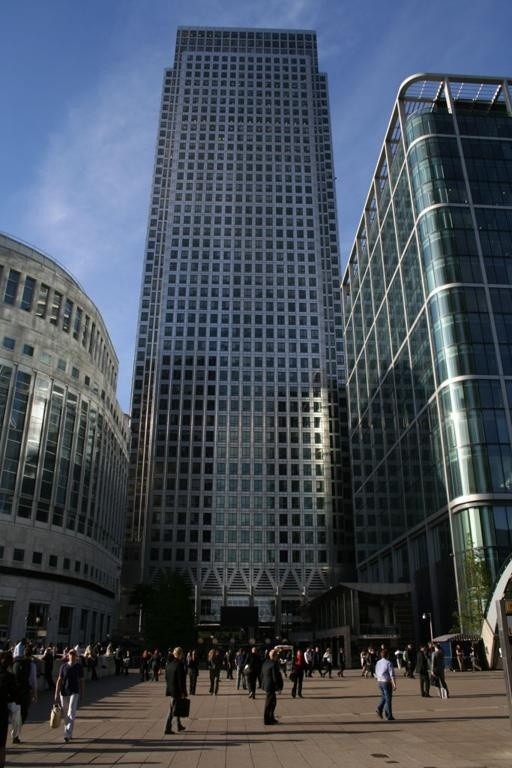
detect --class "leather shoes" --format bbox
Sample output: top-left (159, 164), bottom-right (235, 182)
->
top-left (165, 729), bottom-right (175, 734)
top-left (177, 725), bottom-right (186, 732)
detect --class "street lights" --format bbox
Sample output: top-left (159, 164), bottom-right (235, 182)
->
top-left (137, 600), bottom-right (145, 631)
top-left (421, 611), bottom-right (434, 644)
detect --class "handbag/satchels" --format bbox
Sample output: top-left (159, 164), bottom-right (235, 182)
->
top-left (172, 696), bottom-right (190, 718)
top-left (430, 674), bottom-right (439, 687)
top-left (49, 703), bottom-right (62, 729)
